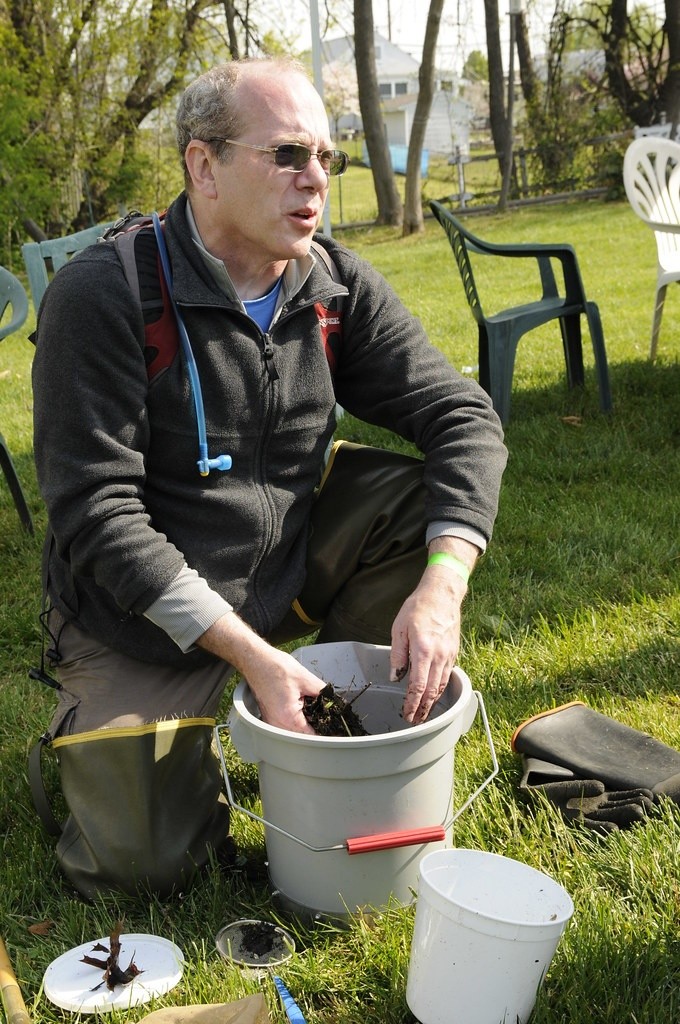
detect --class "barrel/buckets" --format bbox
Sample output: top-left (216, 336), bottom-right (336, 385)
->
top-left (215, 641), bottom-right (499, 913)
top-left (406, 849), bottom-right (574, 1024)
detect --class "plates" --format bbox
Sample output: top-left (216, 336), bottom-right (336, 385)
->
top-left (43, 933), bottom-right (185, 1014)
top-left (214, 919), bottom-right (296, 966)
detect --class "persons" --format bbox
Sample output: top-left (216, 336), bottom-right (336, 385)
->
top-left (25, 54), bottom-right (511, 910)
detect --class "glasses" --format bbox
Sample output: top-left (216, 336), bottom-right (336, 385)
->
top-left (205, 135), bottom-right (352, 178)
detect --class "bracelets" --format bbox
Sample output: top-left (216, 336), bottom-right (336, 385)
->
top-left (427, 552), bottom-right (470, 585)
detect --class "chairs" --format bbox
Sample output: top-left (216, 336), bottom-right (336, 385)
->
top-left (621, 135), bottom-right (680, 364)
top-left (1, 268), bottom-right (37, 542)
top-left (427, 197), bottom-right (613, 432)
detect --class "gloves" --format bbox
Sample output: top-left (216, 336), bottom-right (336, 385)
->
top-left (511, 701), bottom-right (680, 810)
top-left (517, 752), bottom-right (656, 842)
